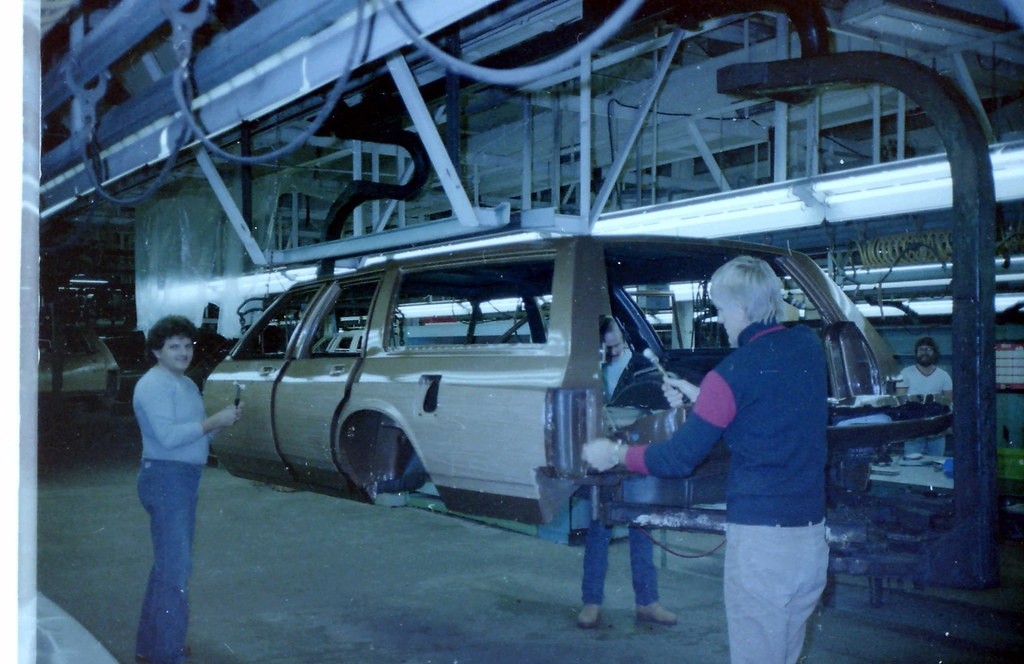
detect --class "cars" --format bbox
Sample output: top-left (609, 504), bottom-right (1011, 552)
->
top-left (205, 231), bottom-right (900, 539)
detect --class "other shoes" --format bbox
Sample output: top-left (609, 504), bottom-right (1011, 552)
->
top-left (578, 604), bottom-right (599, 628)
top-left (636, 602), bottom-right (679, 625)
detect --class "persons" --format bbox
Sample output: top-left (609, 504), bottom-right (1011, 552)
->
top-left (133, 315), bottom-right (243, 664)
top-left (578, 317), bottom-right (677, 628)
top-left (895, 337), bottom-right (953, 457)
top-left (581, 254), bottom-right (830, 664)
